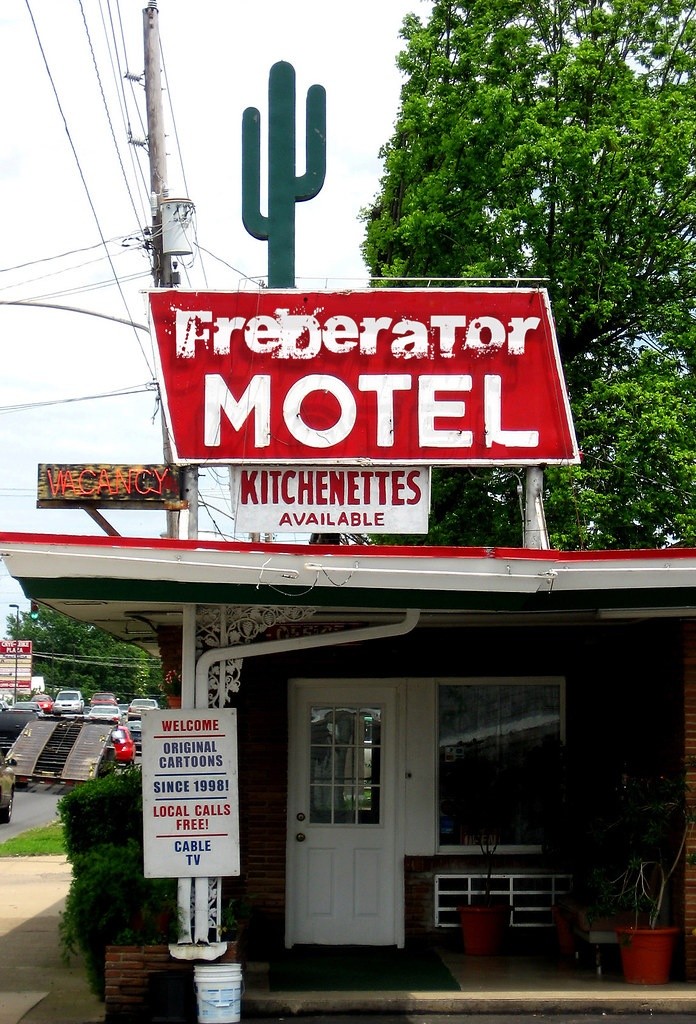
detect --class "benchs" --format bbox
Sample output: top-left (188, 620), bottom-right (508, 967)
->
top-left (557, 862), bottom-right (659, 934)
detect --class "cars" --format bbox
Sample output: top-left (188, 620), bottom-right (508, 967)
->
top-left (88, 693), bottom-right (120, 706)
top-left (127, 699), bottom-right (160, 721)
top-left (0, 701), bottom-right (10, 712)
top-left (52, 691), bottom-right (85, 715)
top-left (29, 694), bottom-right (54, 713)
top-left (11, 702), bottom-right (44, 716)
top-left (111, 727), bottom-right (138, 768)
top-left (125, 720), bottom-right (142, 752)
top-left (89, 706), bottom-right (123, 726)
top-left (117, 703), bottom-right (130, 715)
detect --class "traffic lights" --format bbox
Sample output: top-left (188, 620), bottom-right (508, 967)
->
top-left (30, 600), bottom-right (39, 621)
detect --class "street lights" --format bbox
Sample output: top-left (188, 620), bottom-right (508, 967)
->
top-left (8, 604), bottom-right (20, 705)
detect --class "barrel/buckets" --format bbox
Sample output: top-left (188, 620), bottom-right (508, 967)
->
top-left (194, 962), bottom-right (243, 1023)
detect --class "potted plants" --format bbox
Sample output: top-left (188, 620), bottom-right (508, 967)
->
top-left (453, 832), bottom-right (515, 956)
top-left (599, 753), bottom-right (696, 985)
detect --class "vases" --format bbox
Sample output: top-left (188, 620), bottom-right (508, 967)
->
top-left (167, 697), bottom-right (180, 709)
top-left (552, 906), bottom-right (576, 955)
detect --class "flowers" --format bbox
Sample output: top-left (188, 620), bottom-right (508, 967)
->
top-left (157, 670), bottom-right (182, 696)
